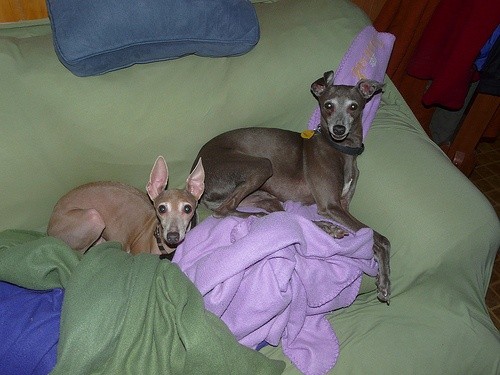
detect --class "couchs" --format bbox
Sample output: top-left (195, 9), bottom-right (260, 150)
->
top-left (0, 0), bottom-right (500, 375)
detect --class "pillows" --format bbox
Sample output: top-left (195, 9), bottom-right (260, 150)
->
top-left (45, 0), bottom-right (260, 77)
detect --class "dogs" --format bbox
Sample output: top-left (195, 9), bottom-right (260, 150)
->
top-left (189, 70), bottom-right (392, 306)
top-left (46, 154), bottom-right (206, 255)
